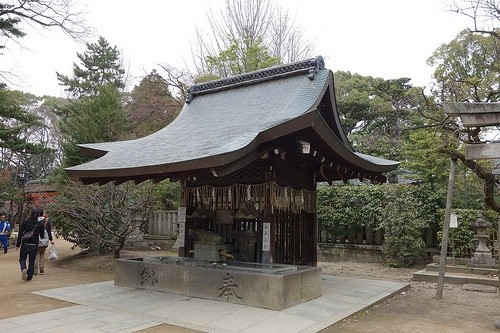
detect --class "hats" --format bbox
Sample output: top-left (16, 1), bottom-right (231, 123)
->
top-left (32, 209), bottom-right (40, 215)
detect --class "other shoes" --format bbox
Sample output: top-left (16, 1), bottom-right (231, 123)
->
top-left (40, 267), bottom-right (44, 273)
top-left (22, 269), bottom-right (27, 280)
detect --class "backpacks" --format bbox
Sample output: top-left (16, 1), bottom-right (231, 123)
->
top-left (38, 229), bottom-right (49, 247)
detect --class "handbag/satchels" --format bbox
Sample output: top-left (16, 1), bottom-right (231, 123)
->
top-left (22, 223), bottom-right (38, 242)
top-left (48, 245), bottom-right (58, 265)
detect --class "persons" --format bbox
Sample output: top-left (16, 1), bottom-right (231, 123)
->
top-left (0, 209), bottom-right (55, 280)
